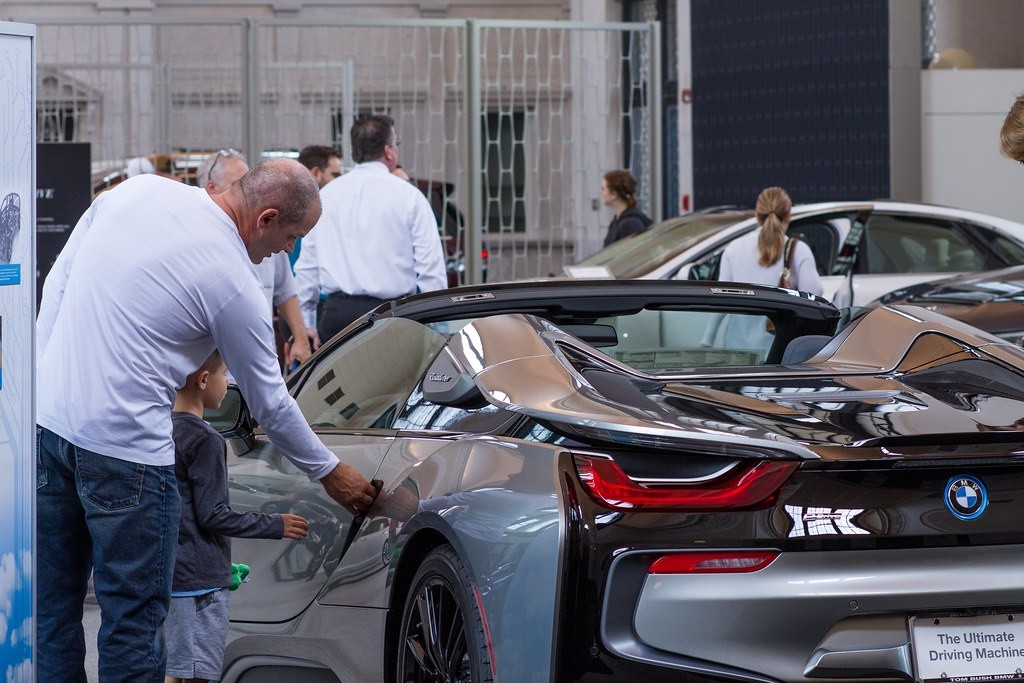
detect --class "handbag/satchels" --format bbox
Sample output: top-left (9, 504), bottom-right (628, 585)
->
top-left (766, 237), bottom-right (801, 336)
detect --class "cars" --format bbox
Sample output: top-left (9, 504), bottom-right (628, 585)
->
top-left (851, 266), bottom-right (1024, 350)
top-left (554, 200), bottom-right (1024, 300)
top-left (224, 475), bottom-right (292, 514)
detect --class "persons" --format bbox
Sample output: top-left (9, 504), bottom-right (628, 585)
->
top-left (601, 170), bottom-right (654, 248)
top-left (35, 115), bottom-right (449, 683)
top-left (701, 187), bottom-right (824, 364)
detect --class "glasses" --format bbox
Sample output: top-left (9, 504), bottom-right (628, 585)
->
top-left (396, 137), bottom-right (399, 145)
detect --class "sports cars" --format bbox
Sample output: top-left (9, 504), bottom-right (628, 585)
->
top-left (202, 279), bottom-right (1024, 683)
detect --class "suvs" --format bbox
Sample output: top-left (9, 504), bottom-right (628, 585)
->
top-left (88, 147), bottom-right (490, 288)
